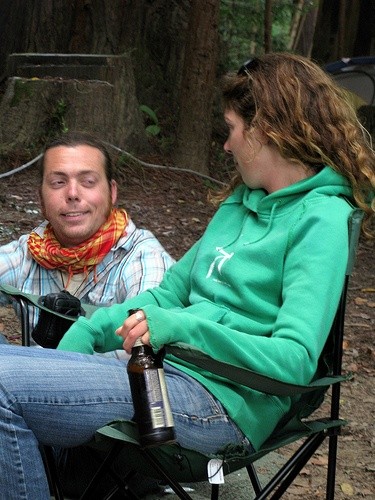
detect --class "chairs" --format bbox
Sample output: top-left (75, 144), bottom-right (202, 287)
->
top-left (0, 207), bottom-right (366, 500)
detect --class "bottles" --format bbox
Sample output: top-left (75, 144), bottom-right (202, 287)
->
top-left (128, 308), bottom-right (176, 449)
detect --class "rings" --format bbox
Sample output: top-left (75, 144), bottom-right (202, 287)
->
top-left (135, 312), bottom-right (143, 322)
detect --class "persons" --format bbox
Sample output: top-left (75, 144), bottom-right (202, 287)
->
top-left (0, 131), bottom-right (176, 348)
top-left (0, 52), bottom-right (375, 500)
